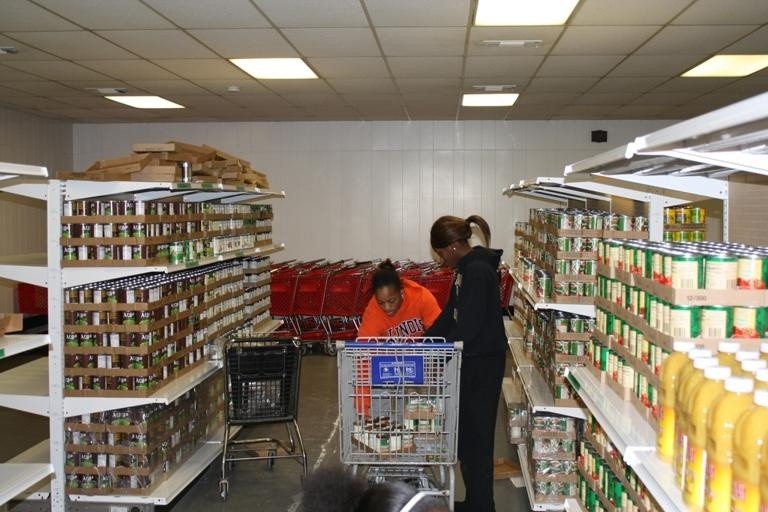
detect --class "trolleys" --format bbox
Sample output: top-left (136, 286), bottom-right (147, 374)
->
top-left (212, 327), bottom-right (316, 500)
top-left (256, 256), bottom-right (514, 357)
top-left (330, 331), bottom-right (469, 508)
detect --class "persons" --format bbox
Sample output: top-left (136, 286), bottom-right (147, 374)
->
top-left (353, 263), bottom-right (442, 415)
top-left (420, 215), bottom-right (507, 512)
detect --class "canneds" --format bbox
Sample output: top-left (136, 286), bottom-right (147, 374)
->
top-left (62, 197), bottom-right (281, 265)
top-left (662, 204), bottom-right (704, 244)
top-left (506, 206), bottom-right (647, 406)
top-left (62, 253), bottom-right (275, 395)
top-left (62, 368), bottom-right (226, 491)
top-left (351, 399), bottom-right (443, 457)
top-left (587, 240), bottom-right (768, 416)
top-left (510, 408), bottom-right (576, 503)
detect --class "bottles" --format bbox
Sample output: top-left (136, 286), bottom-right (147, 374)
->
top-left (656, 340), bottom-right (768, 512)
